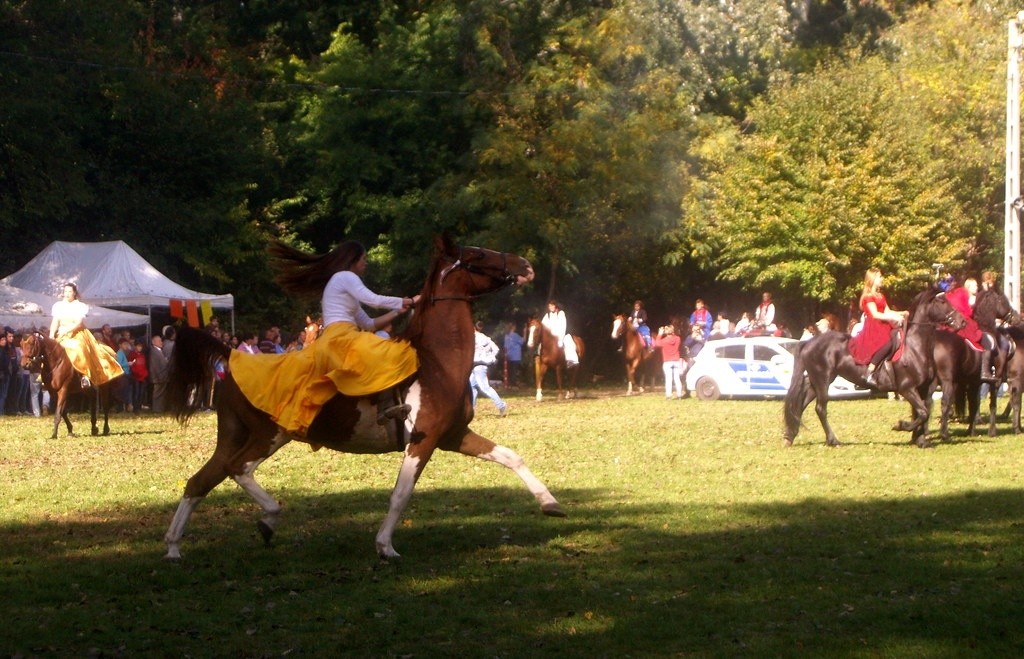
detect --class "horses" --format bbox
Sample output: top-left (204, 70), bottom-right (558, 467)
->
top-left (923, 315), bottom-right (1024, 438)
top-left (611, 312), bottom-right (664, 393)
top-left (668, 312), bottom-right (692, 338)
top-left (163, 232), bottom-right (568, 561)
top-left (19, 330), bottom-right (112, 439)
top-left (527, 317), bottom-right (586, 400)
top-left (783, 282), bottom-right (967, 448)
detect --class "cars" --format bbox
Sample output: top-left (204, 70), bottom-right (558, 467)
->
top-left (685, 335), bottom-right (871, 401)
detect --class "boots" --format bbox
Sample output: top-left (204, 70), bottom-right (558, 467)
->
top-left (979, 349), bottom-right (998, 381)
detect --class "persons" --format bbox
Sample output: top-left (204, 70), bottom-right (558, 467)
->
top-left (0, 326), bottom-right (177, 417)
top-left (49, 283), bottom-right (125, 388)
top-left (654, 325), bottom-right (684, 400)
top-left (617, 299), bottom-right (653, 353)
top-left (320, 238), bottom-right (420, 426)
top-left (469, 321), bottom-right (506, 418)
top-left (375, 322), bottom-right (393, 339)
top-left (683, 266), bottom-right (1001, 384)
top-left (505, 321), bottom-right (528, 389)
top-left (541, 300), bottom-right (579, 368)
top-left (208, 312), bottom-right (325, 405)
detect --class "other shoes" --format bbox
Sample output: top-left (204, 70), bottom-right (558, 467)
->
top-left (42, 405), bottom-right (49, 416)
top-left (115, 407), bottom-right (125, 414)
top-left (567, 361), bottom-right (574, 368)
top-left (861, 374), bottom-right (878, 385)
top-left (500, 402), bottom-right (507, 416)
top-left (10, 409), bottom-right (34, 416)
top-left (133, 407), bottom-right (140, 415)
top-left (377, 403), bottom-right (412, 425)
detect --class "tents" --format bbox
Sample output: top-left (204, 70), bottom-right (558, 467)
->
top-left (0, 240), bottom-right (235, 348)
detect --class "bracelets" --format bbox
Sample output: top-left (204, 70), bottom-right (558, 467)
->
top-left (410, 296), bottom-right (414, 306)
